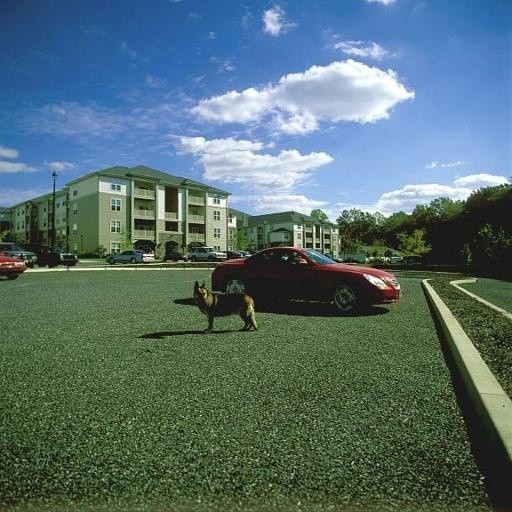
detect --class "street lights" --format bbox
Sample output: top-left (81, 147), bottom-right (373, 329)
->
top-left (381, 239), bottom-right (387, 255)
top-left (51, 171), bottom-right (59, 244)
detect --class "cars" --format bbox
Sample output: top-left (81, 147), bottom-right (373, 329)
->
top-left (106, 250), bottom-right (154, 264)
top-left (238, 251), bottom-right (252, 258)
top-left (1, 243), bottom-right (38, 269)
top-left (225, 250), bottom-right (243, 260)
top-left (211, 247), bottom-right (401, 312)
top-left (332, 255), bottom-right (400, 263)
top-left (164, 252), bottom-right (188, 262)
top-left (0, 251), bottom-right (27, 279)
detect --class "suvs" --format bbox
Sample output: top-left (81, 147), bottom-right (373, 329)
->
top-left (189, 247), bottom-right (227, 262)
top-left (23, 244), bottom-right (79, 268)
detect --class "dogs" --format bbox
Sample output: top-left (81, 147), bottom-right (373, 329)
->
top-left (194, 280), bottom-right (257, 333)
